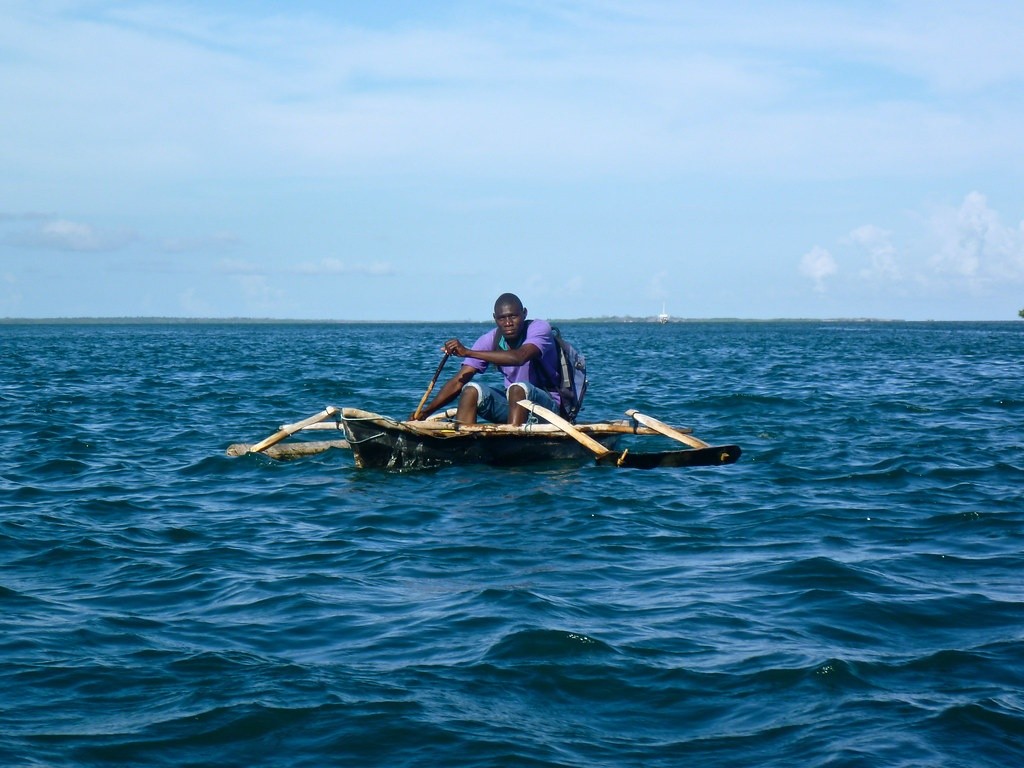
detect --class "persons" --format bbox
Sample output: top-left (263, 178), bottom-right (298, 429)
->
top-left (407, 293), bottom-right (565, 426)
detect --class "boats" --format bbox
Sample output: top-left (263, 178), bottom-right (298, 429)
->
top-left (226, 399), bottom-right (742, 475)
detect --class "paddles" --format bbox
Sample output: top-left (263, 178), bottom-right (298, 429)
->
top-left (413, 352), bottom-right (449, 420)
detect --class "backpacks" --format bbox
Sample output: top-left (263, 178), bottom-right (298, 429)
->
top-left (493, 319), bottom-right (589, 424)
